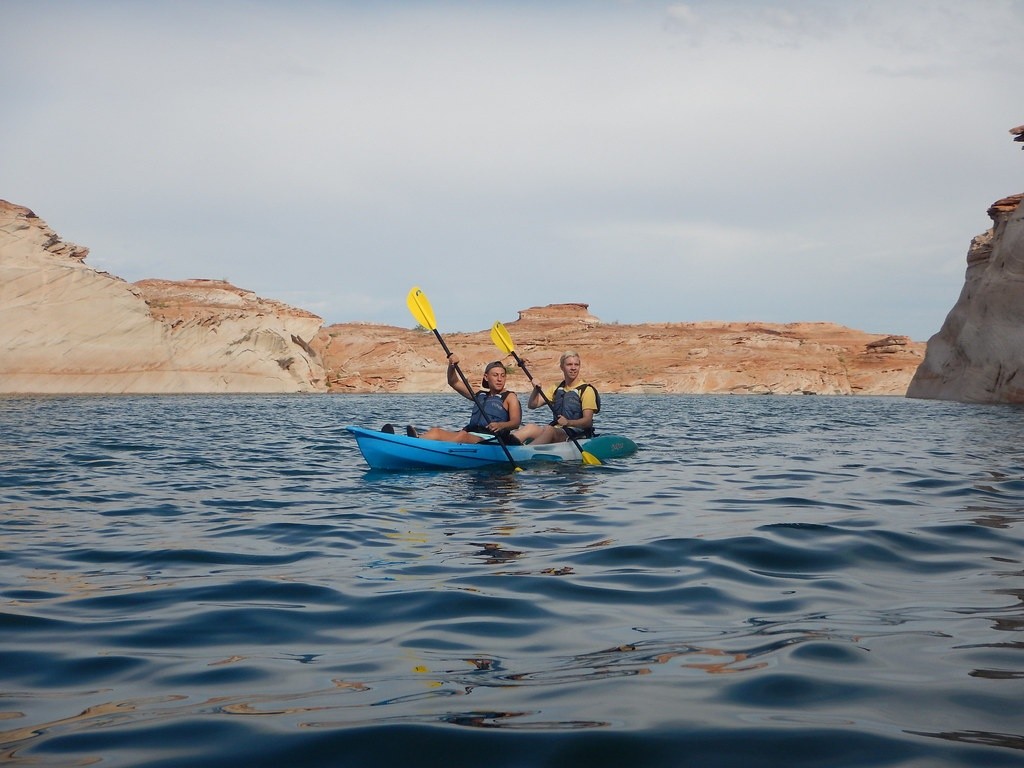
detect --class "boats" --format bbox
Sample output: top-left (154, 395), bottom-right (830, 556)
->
top-left (346, 424), bottom-right (640, 474)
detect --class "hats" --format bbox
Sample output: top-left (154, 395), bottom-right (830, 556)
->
top-left (482, 361), bottom-right (506, 389)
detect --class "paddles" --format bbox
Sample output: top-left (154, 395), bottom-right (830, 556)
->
top-left (490, 318), bottom-right (602, 465)
top-left (407, 286), bottom-right (526, 472)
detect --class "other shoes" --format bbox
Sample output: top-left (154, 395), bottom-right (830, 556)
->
top-left (381, 423), bottom-right (394, 434)
top-left (407, 425), bottom-right (419, 438)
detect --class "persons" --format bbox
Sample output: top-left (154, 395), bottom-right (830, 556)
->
top-left (509, 350), bottom-right (601, 445)
top-left (381, 353), bottom-right (522, 444)
